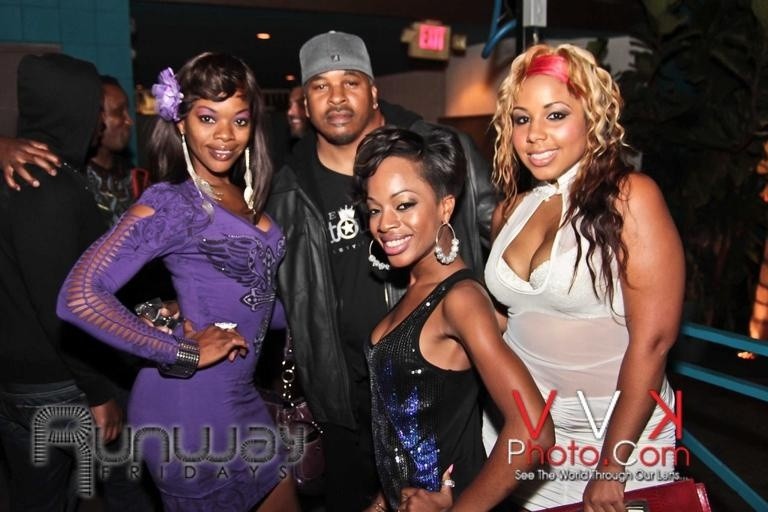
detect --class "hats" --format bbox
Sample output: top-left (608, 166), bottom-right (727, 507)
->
top-left (299, 30), bottom-right (374, 87)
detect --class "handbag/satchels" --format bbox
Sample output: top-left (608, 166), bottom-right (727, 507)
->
top-left (257, 327), bottom-right (326, 487)
top-left (534, 478), bottom-right (712, 512)
